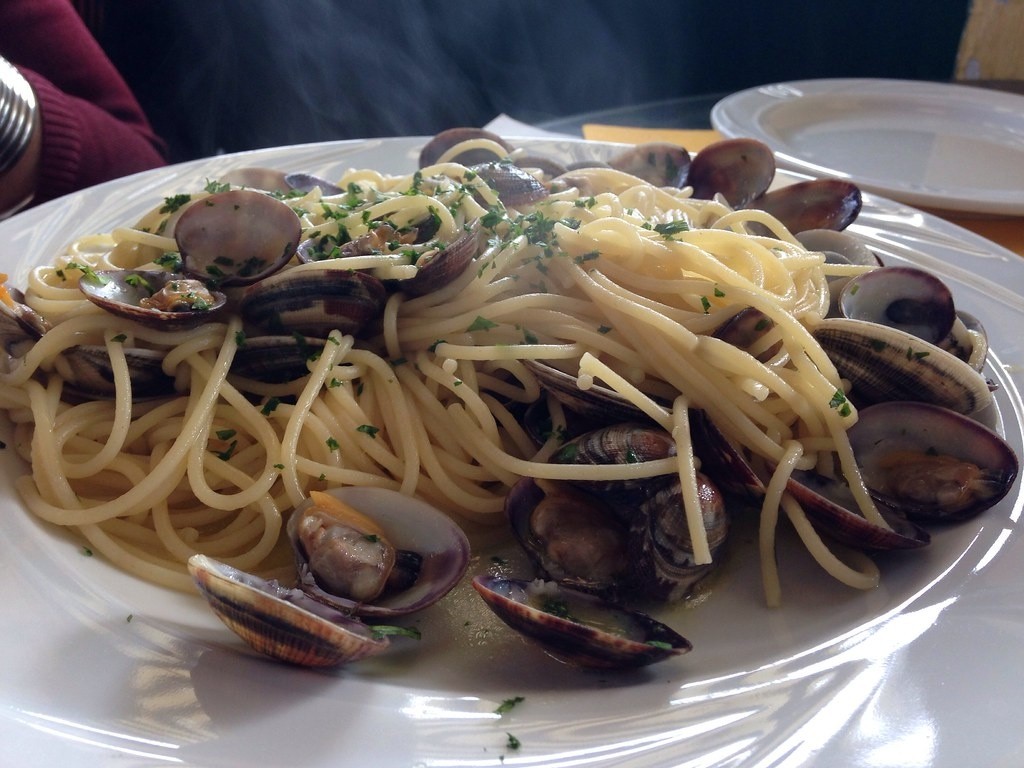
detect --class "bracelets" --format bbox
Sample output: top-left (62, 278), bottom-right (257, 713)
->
top-left (0, 54), bottom-right (37, 174)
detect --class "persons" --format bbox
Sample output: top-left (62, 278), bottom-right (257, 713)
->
top-left (0, 0), bottom-right (171, 225)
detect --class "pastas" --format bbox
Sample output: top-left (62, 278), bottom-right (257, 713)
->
top-left (0, 138), bottom-right (1023, 608)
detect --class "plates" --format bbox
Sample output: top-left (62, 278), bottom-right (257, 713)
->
top-left (0, 135), bottom-right (1024, 768)
top-left (710, 78), bottom-right (1024, 220)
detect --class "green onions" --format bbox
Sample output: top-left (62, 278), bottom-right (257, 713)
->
top-left (58, 155), bottom-right (929, 750)
